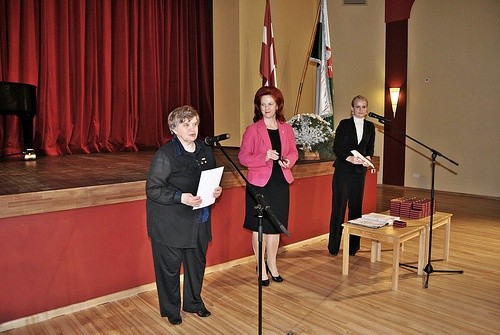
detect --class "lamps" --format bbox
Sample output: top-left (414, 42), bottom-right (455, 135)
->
top-left (390, 88), bottom-right (400, 117)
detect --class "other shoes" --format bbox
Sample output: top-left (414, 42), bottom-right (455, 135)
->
top-left (329, 249), bottom-right (338, 255)
top-left (349, 251), bottom-right (356, 255)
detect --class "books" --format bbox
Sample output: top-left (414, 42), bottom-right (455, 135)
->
top-left (347, 213), bottom-right (401, 229)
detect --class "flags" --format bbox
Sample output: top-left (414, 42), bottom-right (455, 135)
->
top-left (260, 0), bottom-right (278, 87)
top-left (309, 0), bottom-right (334, 131)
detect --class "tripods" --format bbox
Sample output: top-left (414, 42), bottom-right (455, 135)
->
top-left (379, 119), bottom-right (465, 288)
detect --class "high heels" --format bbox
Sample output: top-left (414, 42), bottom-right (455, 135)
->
top-left (265, 261), bottom-right (283, 282)
top-left (256, 266), bottom-right (269, 286)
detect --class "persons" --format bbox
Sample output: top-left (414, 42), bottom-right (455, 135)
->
top-left (145, 106), bottom-right (222, 325)
top-left (328, 95), bottom-right (376, 256)
top-left (237, 86), bottom-right (299, 286)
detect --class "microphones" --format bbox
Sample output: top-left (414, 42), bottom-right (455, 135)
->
top-left (205, 133), bottom-right (231, 145)
top-left (369, 112), bottom-right (392, 123)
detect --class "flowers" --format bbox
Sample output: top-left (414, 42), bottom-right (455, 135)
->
top-left (286, 113), bottom-right (334, 152)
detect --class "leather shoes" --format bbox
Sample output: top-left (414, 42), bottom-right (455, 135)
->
top-left (168, 316), bottom-right (182, 325)
top-left (196, 309), bottom-right (211, 316)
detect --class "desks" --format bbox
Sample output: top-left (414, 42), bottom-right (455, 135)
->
top-left (341, 210), bottom-right (453, 291)
